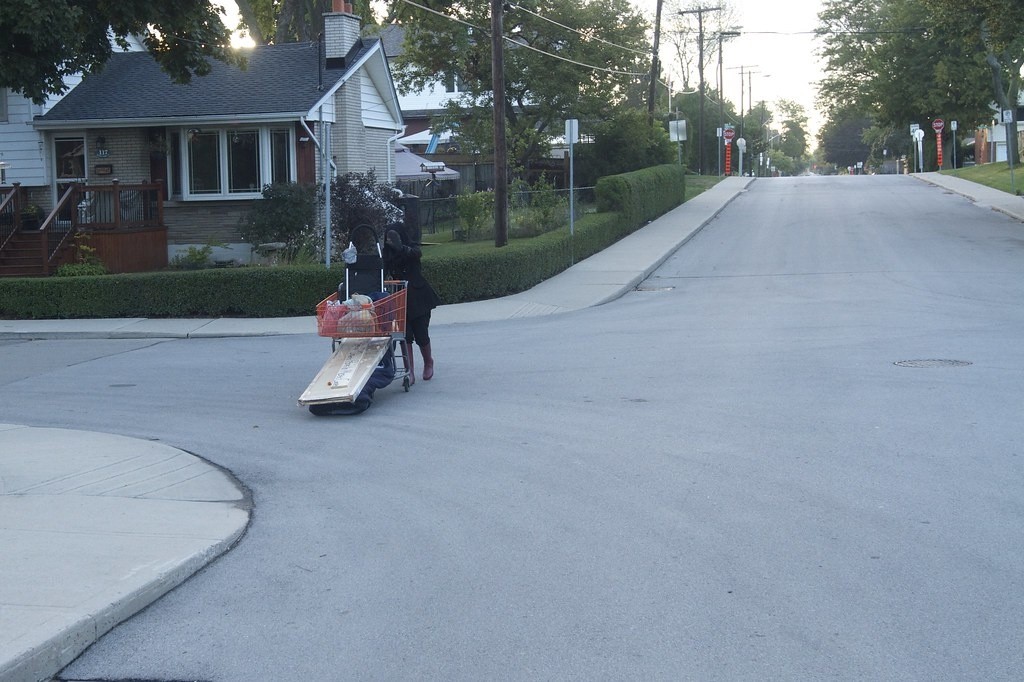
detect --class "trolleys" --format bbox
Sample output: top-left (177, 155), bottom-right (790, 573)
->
top-left (315, 278), bottom-right (412, 392)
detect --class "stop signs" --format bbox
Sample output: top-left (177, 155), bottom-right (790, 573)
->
top-left (932, 119), bottom-right (944, 132)
top-left (724, 129), bottom-right (735, 139)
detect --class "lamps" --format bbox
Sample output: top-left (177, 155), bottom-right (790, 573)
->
top-left (95, 135), bottom-right (106, 149)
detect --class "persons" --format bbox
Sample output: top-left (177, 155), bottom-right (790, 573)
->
top-left (382, 224), bottom-right (439, 387)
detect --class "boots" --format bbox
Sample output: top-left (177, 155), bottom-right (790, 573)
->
top-left (400, 343), bottom-right (415, 387)
top-left (419, 343), bottom-right (433, 380)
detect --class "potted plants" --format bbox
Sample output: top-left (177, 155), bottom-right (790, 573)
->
top-left (19, 203), bottom-right (45, 223)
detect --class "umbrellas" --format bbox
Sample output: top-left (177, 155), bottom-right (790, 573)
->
top-left (395, 141), bottom-right (459, 181)
top-left (398, 122), bottom-right (461, 144)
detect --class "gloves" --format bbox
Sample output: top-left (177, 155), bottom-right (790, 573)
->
top-left (386, 229), bottom-right (404, 252)
